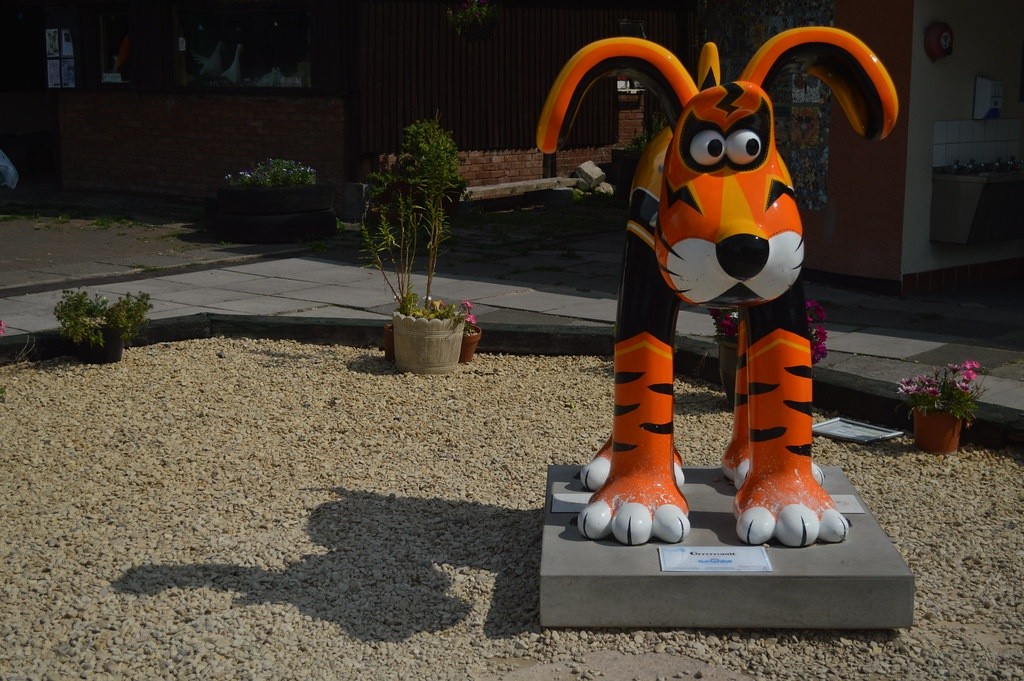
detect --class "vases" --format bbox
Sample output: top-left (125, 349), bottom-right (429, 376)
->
top-left (382, 325), bottom-right (395, 362)
top-left (460, 325), bottom-right (481, 362)
top-left (913, 409), bottom-right (962, 453)
top-left (716, 339), bottom-right (738, 405)
top-left (464, 22), bottom-right (494, 40)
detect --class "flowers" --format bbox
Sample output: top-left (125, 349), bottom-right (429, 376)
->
top-left (709, 299), bottom-right (827, 365)
top-left (445, 0), bottom-right (500, 36)
top-left (460, 301), bottom-right (477, 334)
top-left (895, 360), bottom-right (988, 427)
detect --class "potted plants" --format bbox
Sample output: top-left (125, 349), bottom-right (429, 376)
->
top-left (53, 290), bottom-right (153, 365)
top-left (360, 120), bottom-right (465, 376)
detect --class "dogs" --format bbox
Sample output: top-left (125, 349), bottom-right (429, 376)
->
top-left (534, 24), bottom-right (900, 548)
